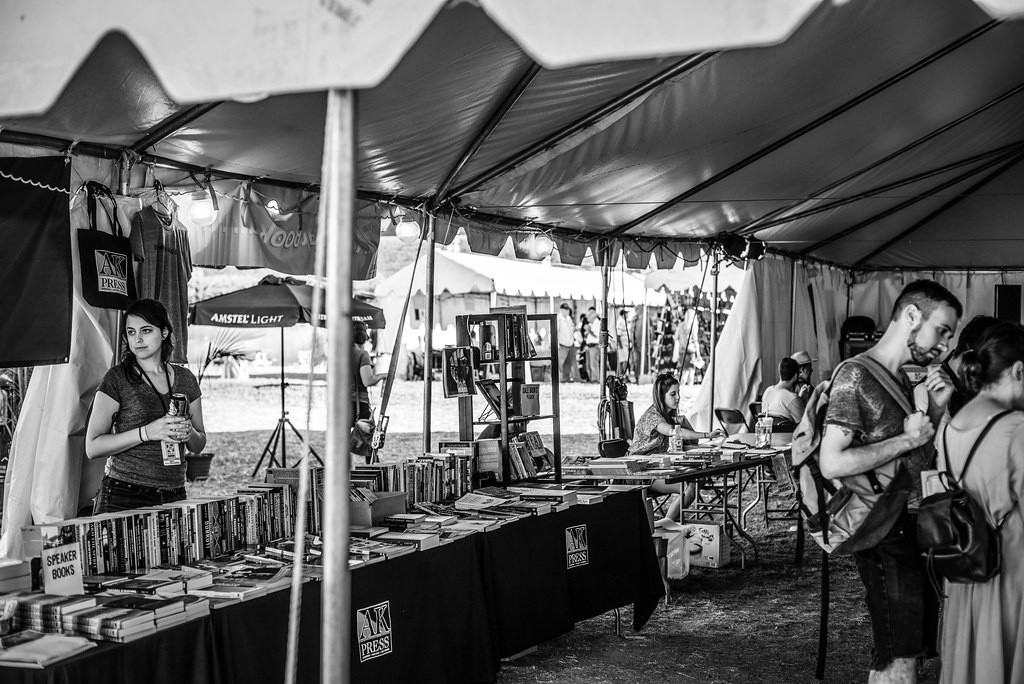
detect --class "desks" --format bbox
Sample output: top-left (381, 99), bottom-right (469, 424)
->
top-left (0, 485), bottom-right (666, 684)
top-left (557, 446), bottom-right (780, 572)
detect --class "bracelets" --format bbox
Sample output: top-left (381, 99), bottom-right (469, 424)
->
top-left (139, 426), bottom-right (144, 443)
top-left (145, 425), bottom-right (150, 441)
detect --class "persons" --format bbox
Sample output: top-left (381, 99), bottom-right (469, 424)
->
top-left (349, 320), bottom-right (394, 464)
top-left (762, 350), bottom-right (815, 425)
top-left (674, 308), bottom-right (700, 387)
top-left (816, 279), bottom-right (1024, 684)
top-left (85, 298), bottom-right (206, 516)
top-left (558, 303), bottom-right (629, 383)
top-left (626, 373), bottom-right (728, 521)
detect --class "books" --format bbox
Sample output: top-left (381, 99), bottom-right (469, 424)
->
top-left (0, 432), bottom-right (650, 668)
top-left (627, 439), bottom-right (793, 476)
top-left (443, 305), bottom-right (540, 420)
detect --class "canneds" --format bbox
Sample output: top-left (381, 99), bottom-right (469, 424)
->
top-left (167, 393), bottom-right (188, 417)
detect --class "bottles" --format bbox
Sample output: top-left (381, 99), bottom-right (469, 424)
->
top-left (755, 418), bottom-right (765, 448)
top-left (669, 425), bottom-right (683, 452)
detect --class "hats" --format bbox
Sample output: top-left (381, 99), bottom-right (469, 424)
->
top-left (790, 350), bottom-right (818, 366)
top-left (561, 303), bottom-right (569, 309)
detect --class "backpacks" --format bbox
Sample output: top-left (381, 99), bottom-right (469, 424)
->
top-left (790, 355), bottom-right (927, 556)
top-left (915, 411), bottom-right (1022, 584)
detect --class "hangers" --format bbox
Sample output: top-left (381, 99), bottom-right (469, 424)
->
top-left (151, 180), bottom-right (172, 215)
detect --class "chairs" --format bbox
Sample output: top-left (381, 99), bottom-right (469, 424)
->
top-left (715, 403), bottom-right (797, 496)
top-left (598, 438), bottom-right (669, 520)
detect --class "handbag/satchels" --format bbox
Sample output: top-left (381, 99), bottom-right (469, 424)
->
top-left (349, 421), bottom-right (376, 457)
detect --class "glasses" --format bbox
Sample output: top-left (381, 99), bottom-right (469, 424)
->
top-left (655, 372), bottom-right (672, 384)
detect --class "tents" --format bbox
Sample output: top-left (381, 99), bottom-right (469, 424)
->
top-left (1, 1), bottom-right (1023, 684)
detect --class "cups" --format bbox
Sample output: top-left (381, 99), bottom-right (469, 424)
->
top-left (762, 418), bottom-right (774, 446)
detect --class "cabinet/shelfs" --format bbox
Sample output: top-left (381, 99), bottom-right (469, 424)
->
top-left (456, 313), bottom-right (561, 491)
